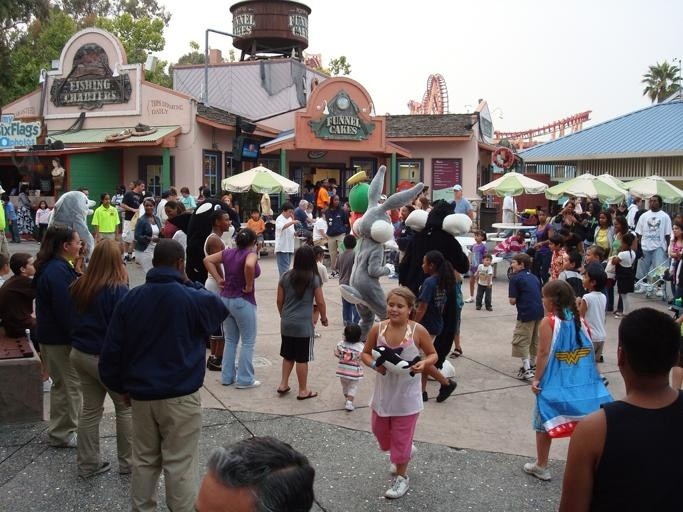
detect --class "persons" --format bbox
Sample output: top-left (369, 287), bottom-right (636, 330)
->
top-left (2, 180), bottom-right (265, 389)
top-left (494, 194), bottom-right (681, 394)
top-left (559, 307), bottom-right (681, 512)
top-left (189, 436), bottom-right (316, 512)
top-left (99, 238), bottom-right (231, 511)
top-left (524, 279), bottom-right (614, 481)
top-left (274, 176), bottom-right (494, 403)
top-left (62, 238), bottom-right (135, 479)
top-left (359, 286), bottom-right (438, 498)
top-left (32, 225), bottom-right (84, 448)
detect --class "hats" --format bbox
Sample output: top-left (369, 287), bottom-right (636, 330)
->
top-left (453, 184), bottom-right (462, 190)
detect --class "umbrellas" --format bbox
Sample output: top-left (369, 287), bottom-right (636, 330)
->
top-left (478, 171), bottom-right (626, 223)
top-left (221, 162), bottom-right (301, 216)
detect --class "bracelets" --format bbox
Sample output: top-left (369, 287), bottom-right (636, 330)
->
top-left (372, 361), bottom-right (376, 367)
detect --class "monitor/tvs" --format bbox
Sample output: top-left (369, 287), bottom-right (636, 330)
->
top-left (241, 138), bottom-right (260, 161)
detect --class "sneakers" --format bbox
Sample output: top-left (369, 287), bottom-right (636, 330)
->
top-left (436, 379), bottom-right (457, 402)
top-left (53, 434), bottom-right (80, 448)
top-left (513, 364), bottom-right (537, 380)
top-left (385, 474), bottom-right (410, 500)
top-left (206, 356), bottom-right (223, 372)
top-left (422, 390), bottom-right (429, 402)
top-left (612, 308), bottom-right (626, 321)
top-left (235, 380), bottom-right (262, 389)
top-left (464, 298), bottom-right (493, 311)
top-left (389, 445), bottom-right (417, 473)
top-left (123, 253), bottom-right (135, 263)
top-left (328, 270), bottom-right (340, 279)
top-left (523, 462), bottom-right (553, 481)
top-left (80, 461), bottom-right (110, 477)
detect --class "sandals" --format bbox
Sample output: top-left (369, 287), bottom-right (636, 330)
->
top-left (450, 347), bottom-right (463, 360)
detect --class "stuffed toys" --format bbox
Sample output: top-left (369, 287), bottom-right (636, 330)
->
top-left (48, 191), bottom-right (96, 261)
top-left (371, 346), bottom-right (420, 377)
top-left (338, 165), bottom-right (424, 342)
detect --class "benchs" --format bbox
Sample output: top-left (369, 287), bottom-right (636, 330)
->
top-left (236, 222), bottom-right (311, 259)
top-left (486, 232), bottom-right (533, 242)
top-left (487, 254), bottom-right (504, 279)
top-left (0, 325), bottom-right (44, 424)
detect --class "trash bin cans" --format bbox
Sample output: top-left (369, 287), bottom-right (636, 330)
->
top-left (480, 206), bottom-right (497, 233)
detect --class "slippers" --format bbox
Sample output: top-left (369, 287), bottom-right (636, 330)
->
top-left (277, 385), bottom-right (291, 394)
top-left (296, 390), bottom-right (318, 400)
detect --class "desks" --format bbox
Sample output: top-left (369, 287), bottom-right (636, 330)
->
top-left (492, 222), bottom-right (536, 238)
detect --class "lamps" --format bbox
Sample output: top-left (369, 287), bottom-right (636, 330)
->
top-left (362, 102), bottom-right (375, 117)
top-left (316, 100), bottom-right (331, 115)
top-left (39, 68), bottom-right (47, 84)
top-left (111, 61), bottom-right (123, 77)
